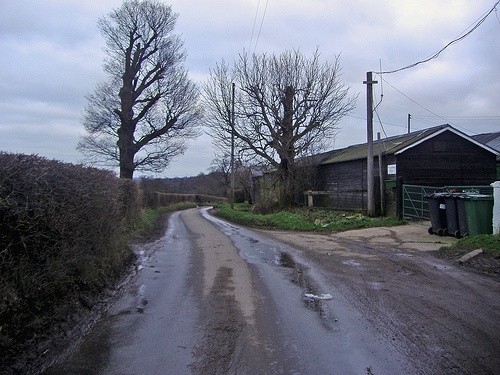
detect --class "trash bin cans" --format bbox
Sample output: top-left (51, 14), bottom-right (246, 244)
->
top-left (424, 193), bottom-right (447, 236)
top-left (463, 194), bottom-right (495, 236)
top-left (443, 193), bottom-right (462, 238)
top-left (455, 195), bottom-right (470, 237)
